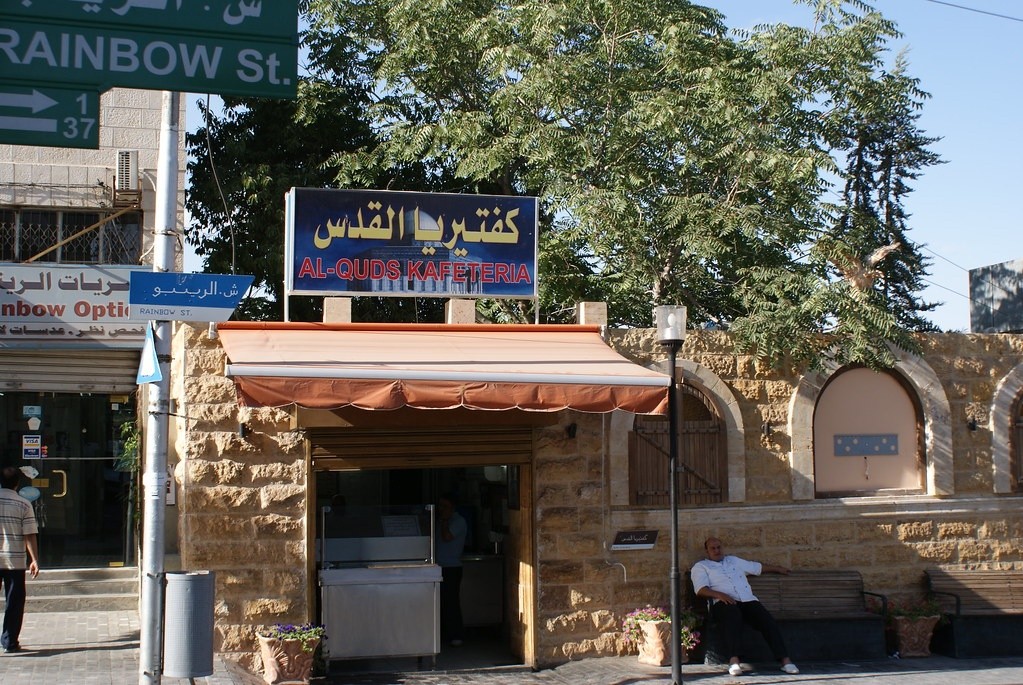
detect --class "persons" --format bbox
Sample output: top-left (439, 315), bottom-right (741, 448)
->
top-left (0, 468), bottom-right (39, 653)
top-left (690, 537), bottom-right (799, 675)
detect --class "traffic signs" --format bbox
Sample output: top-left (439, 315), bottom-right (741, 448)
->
top-left (0, 84), bottom-right (100, 150)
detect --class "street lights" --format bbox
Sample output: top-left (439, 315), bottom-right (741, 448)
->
top-left (657, 305), bottom-right (687, 685)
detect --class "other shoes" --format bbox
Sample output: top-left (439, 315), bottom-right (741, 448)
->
top-left (728, 663), bottom-right (743, 676)
top-left (780, 664), bottom-right (800, 675)
top-left (451, 639), bottom-right (464, 647)
top-left (5, 644), bottom-right (22, 653)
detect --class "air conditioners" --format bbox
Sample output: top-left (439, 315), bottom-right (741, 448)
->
top-left (116, 149), bottom-right (141, 206)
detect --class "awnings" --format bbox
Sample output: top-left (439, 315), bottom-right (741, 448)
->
top-left (216, 321), bottom-right (671, 416)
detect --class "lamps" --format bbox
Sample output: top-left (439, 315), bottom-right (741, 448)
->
top-left (967, 415), bottom-right (976, 431)
top-left (237, 423), bottom-right (252, 437)
top-left (761, 421), bottom-right (772, 437)
top-left (565, 422), bottom-right (578, 437)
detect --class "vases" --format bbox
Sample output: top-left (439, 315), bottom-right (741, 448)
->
top-left (892, 615), bottom-right (940, 659)
top-left (636, 618), bottom-right (691, 666)
top-left (259, 631), bottom-right (319, 685)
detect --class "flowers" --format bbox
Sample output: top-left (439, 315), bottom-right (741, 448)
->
top-left (269, 621), bottom-right (330, 643)
top-left (619, 602), bottom-right (698, 652)
top-left (865, 596), bottom-right (952, 627)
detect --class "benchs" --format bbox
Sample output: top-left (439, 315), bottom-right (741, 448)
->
top-left (684, 568), bottom-right (890, 667)
top-left (923, 570), bottom-right (1023, 657)
